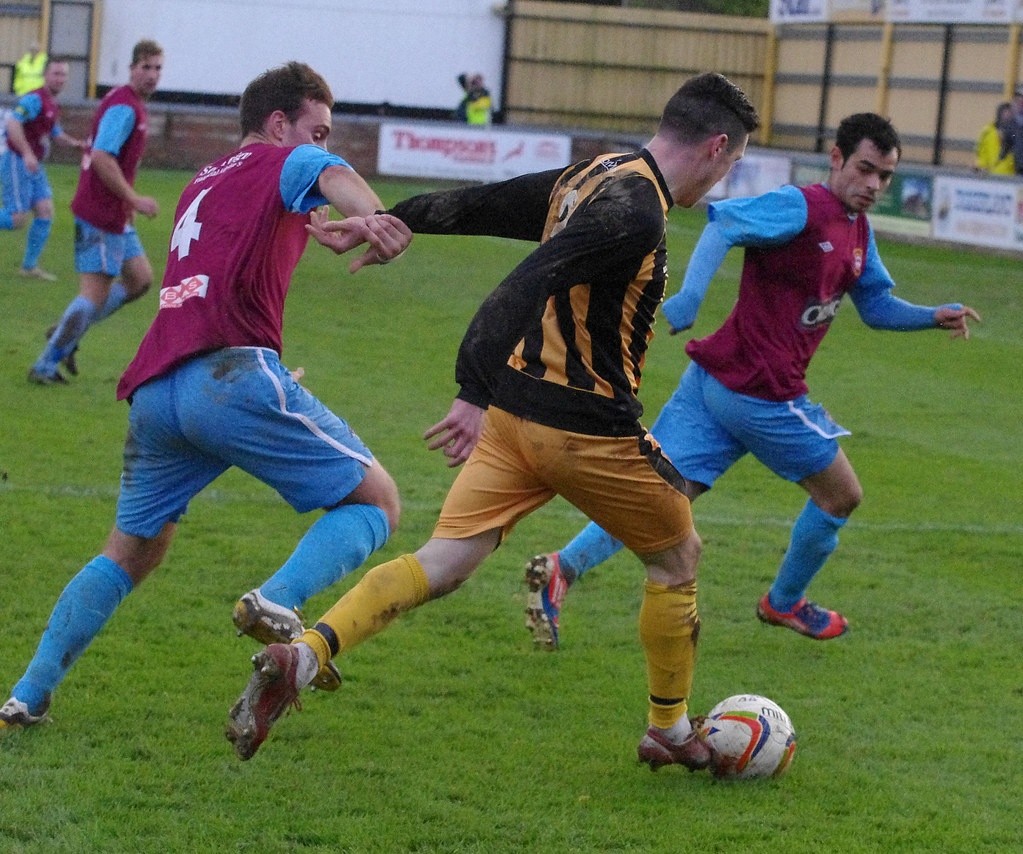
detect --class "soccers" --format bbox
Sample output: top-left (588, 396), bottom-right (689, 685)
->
top-left (698, 694), bottom-right (799, 782)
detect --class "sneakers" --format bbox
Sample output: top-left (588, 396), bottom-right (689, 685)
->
top-left (232, 587), bottom-right (342, 692)
top-left (757, 590), bottom-right (847, 640)
top-left (0, 696), bottom-right (50, 733)
top-left (225, 642), bottom-right (301, 760)
top-left (637, 722), bottom-right (712, 772)
top-left (526, 552), bottom-right (573, 651)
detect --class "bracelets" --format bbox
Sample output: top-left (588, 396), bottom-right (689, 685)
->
top-left (374, 251), bottom-right (390, 263)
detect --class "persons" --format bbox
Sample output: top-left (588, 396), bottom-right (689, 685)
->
top-left (452, 74), bottom-right (493, 124)
top-left (223, 70), bottom-right (763, 775)
top-left (0, 62), bottom-right (415, 731)
top-left (973, 81), bottom-right (1023, 178)
top-left (26, 39), bottom-right (166, 387)
top-left (0, 41), bottom-right (86, 282)
top-left (524, 111), bottom-right (980, 653)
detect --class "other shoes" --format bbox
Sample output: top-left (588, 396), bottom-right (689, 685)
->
top-left (21, 266), bottom-right (55, 281)
top-left (46, 327), bottom-right (79, 377)
top-left (29, 365), bottom-right (68, 386)
